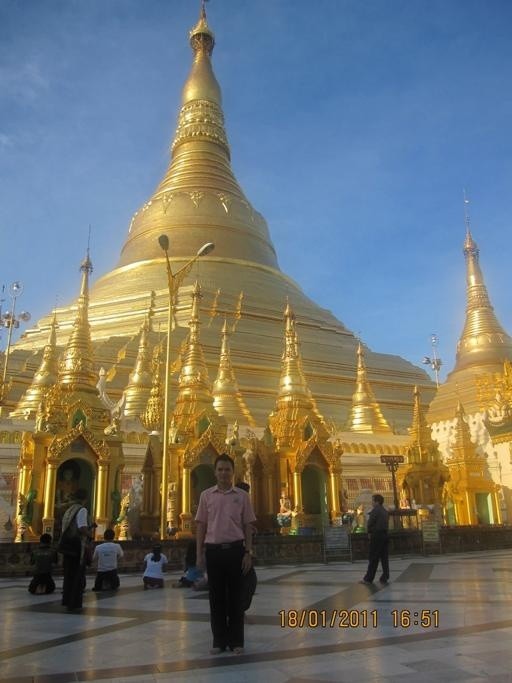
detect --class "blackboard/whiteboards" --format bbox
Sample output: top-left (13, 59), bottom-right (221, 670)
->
top-left (324, 526), bottom-right (350, 549)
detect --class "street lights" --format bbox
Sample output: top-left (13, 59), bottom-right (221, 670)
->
top-left (421, 333), bottom-right (443, 390)
top-left (1, 279), bottom-right (33, 402)
top-left (156, 232), bottom-right (218, 540)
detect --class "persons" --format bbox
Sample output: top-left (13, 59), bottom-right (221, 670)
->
top-left (193, 451), bottom-right (257, 654)
top-left (91, 527), bottom-right (124, 591)
top-left (399, 480), bottom-right (414, 508)
top-left (279, 487), bottom-right (291, 514)
top-left (357, 493), bottom-right (390, 585)
top-left (142, 543), bottom-right (170, 590)
top-left (341, 482), bottom-right (349, 512)
top-left (27, 533), bottom-right (60, 594)
top-left (56, 488), bottom-right (98, 613)
top-left (168, 483), bottom-right (176, 526)
top-left (351, 498), bottom-right (413, 530)
top-left (234, 480), bottom-right (258, 538)
top-left (178, 540), bottom-right (208, 590)
top-left (54, 467), bottom-right (78, 516)
top-left (60, 544), bottom-right (92, 594)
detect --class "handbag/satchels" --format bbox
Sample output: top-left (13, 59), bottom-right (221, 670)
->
top-left (55, 506), bottom-right (83, 555)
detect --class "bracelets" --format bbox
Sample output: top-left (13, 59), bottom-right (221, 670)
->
top-left (245, 549), bottom-right (253, 555)
